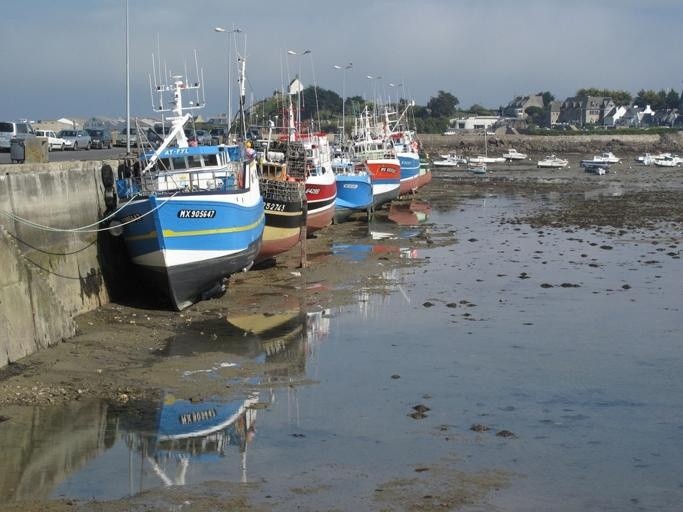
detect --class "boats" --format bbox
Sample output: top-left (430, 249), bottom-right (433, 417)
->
top-left (536, 155), bottom-right (567, 167)
top-left (502, 149), bottom-right (526, 160)
top-left (635, 152), bottom-right (682, 167)
top-left (584, 162), bottom-right (609, 175)
top-left (432, 150), bottom-right (506, 173)
top-left (580, 151), bottom-right (619, 163)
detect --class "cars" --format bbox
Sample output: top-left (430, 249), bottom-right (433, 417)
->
top-left (0, 121), bottom-right (37, 152)
top-left (85, 128), bottom-right (113, 149)
top-left (36, 129), bottom-right (65, 152)
top-left (443, 129), bottom-right (496, 137)
top-left (55, 128), bottom-right (91, 151)
